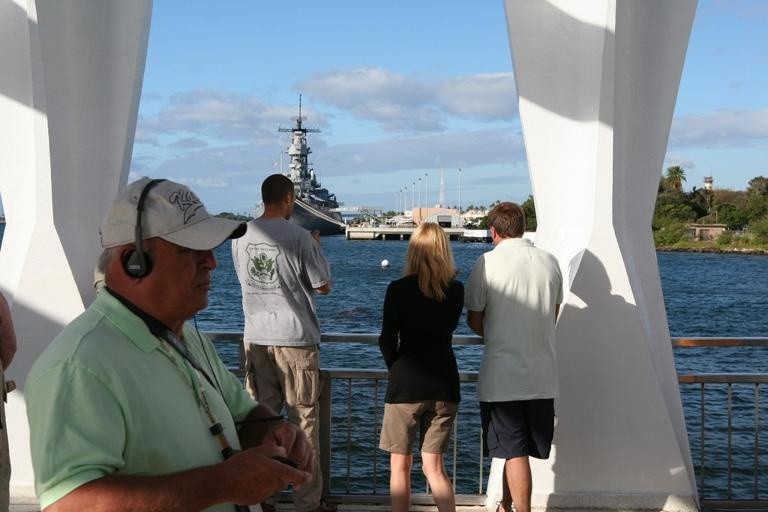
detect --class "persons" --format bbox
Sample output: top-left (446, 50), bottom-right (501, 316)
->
top-left (376, 223), bottom-right (466, 511)
top-left (19, 175), bottom-right (314, 512)
top-left (231, 173), bottom-right (334, 510)
top-left (465, 200), bottom-right (563, 511)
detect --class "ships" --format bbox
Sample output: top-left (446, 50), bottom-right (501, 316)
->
top-left (254, 93), bottom-right (345, 236)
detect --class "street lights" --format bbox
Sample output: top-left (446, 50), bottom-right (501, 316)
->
top-left (424, 172), bottom-right (428, 218)
top-left (392, 189), bottom-right (401, 215)
top-left (417, 177), bottom-right (421, 221)
top-left (403, 186), bottom-right (407, 216)
top-left (456, 168), bottom-right (462, 212)
top-left (411, 182), bottom-right (414, 215)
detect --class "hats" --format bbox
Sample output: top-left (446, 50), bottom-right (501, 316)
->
top-left (100, 177), bottom-right (248, 251)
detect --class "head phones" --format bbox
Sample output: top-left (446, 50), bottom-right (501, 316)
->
top-left (122, 179), bottom-right (166, 278)
